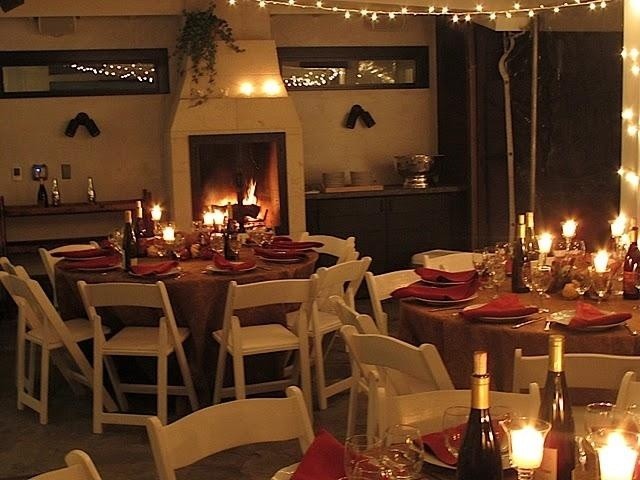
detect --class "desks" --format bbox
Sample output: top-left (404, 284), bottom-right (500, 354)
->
top-left (389, 266), bottom-right (639, 410)
top-left (54, 235), bottom-right (319, 415)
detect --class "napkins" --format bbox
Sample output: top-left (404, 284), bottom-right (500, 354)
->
top-left (50, 246), bottom-right (117, 256)
top-left (211, 252), bottom-right (258, 270)
top-left (390, 279), bottom-right (483, 302)
top-left (129, 260), bottom-right (180, 275)
top-left (413, 418), bottom-right (511, 466)
top-left (255, 246), bottom-right (298, 261)
top-left (56, 256), bottom-right (121, 271)
top-left (569, 300), bottom-right (632, 330)
top-left (414, 266), bottom-right (477, 284)
top-left (270, 237), bottom-right (326, 251)
top-left (460, 292), bottom-right (540, 320)
top-left (289, 425), bottom-right (384, 479)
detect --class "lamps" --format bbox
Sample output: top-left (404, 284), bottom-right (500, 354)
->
top-left (344, 104), bottom-right (378, 129)
top-left (64, 112), bottom-right (100, 138)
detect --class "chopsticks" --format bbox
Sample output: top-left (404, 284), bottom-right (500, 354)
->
top-left (256, 252), bottom-right (301, 264)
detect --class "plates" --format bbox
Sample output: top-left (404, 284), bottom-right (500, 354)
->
top-left (275, 247), bottom-right (313, 252)
top-left (70, 264), bottom-right (120, 273)
top-left (412, 278), bottom-right (626, 331)
top-left (320, 169), bottom-right (369, 189)
top-left (422, 445), bottom-right (457, 470)
top-left (203, 264), bottom-right (258, 273)
top-left (67, 256), bottom-right (103, 261)
top-left (129, 266), bottom-right (179, 279)
top-left (267, 462), bottom-right (302, 479)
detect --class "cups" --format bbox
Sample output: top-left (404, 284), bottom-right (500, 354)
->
top-left (583, 402), bottom-right (622, 436)
top-left (439, 405), bottom-right (473, 459)
top-left (487, 402), bottom-right (518, 471)
top-left (624, 404), bottom-right (640, 432)
top-left (585, 429), bottom-right (640, 480)
top-left (337, 423), bottom-right (426, 480)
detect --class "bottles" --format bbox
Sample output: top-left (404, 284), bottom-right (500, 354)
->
top-left (134, 200), bottom-right (149, 257)
top-left (223, 203), bottom-right (240, 261)
top-left (511, 214), bottom-right (532, 293)
top-left (121, 210), bottom-right (140, 271)
top-left (526, 212), bottom-right (539, 261)
top-left (621, 226), bottom-right (640, 300)
top-left (51, 178), bottom-right (61, 207)
top-left (86, 176), bottom-right (95, 203)
top-left (538, 333), bottom-right (577, 480)
top-left (453, 350), bottom-right (504, 480)
top-left (36, 178), bottom-right (49, 207)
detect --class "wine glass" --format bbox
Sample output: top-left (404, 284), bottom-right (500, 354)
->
top-left (153, 218), bottom-right (274, 259)
top-left (559, 221), bottom-right (580, 255)
top-left (607, 219), bottom-right (626, 255)
top-left (149, 208), bottom-right (164, 234)
top-left (497, 415), bottom-right (551, 480)
top-left (474, 234), bottom-right (617, 305)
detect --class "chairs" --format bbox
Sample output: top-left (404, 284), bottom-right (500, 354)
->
top-left (297, 230), bottom-right (360, 265)
top-left (365, 269), bottom-right (420, 336)
top-left (615, 370), bottom-right (639, 417)
top-left (373, 383), bottom-right (541, 447)
top-left (38, 241), bottom-right (101, 309)
top-left (1, 270), bottom-right (131, 425)
top-left (339, 323), bottom-right (455, 450)
top-left (1, 254), bottom-right (83, 402)
top-left (77, 279), bottom-right (199, 435)
top-left (423, 252), bottom-right (495, 273)
top-left (211, 272), bottom-right (321, 424)
top-left (280, 255), bottom-right (373, 409)
top-left (146, 386), bottom-right (317, 480)
top-left (31, 450), bottom-right (103, 479)
top-left (512, 348), bottom-right (639, 394)
top-left (328, 294), bottom-right (380, 446)
